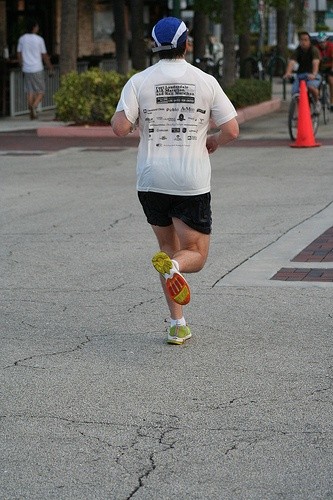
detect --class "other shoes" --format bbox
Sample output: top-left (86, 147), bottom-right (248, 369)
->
top-left (31, 105), bottom-right (38, 119)
top-left (314, 101), bottom-right (322, 115)
top-left (330, 105), bottom-right (333, 110)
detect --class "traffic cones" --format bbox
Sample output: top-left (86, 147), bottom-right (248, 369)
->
top-left (289, 80), bottom-right (321, 148)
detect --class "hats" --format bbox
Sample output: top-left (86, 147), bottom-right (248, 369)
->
top-left (151, 16), bottom-right (189, 52)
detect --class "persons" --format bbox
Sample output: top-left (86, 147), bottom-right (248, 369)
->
top-left (17, 19), bottom-right (55, 119)
top-left (282, 31), bottom-right (324, 129)
top-left (312, 31), bottom-right (333, 112)
top-left (111, 16), bottom-right (239, 344)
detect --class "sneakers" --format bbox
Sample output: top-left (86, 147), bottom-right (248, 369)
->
top-left (152, 250), bottom-right (191, 305)
top-left (166, 324), bottom-right (192, 345)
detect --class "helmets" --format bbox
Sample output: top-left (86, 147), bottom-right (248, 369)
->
top-left (317, 33), bottom-right (328, 43)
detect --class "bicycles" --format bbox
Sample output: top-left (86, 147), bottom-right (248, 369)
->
top-left (284, 74), bottom-right (331, 142)
